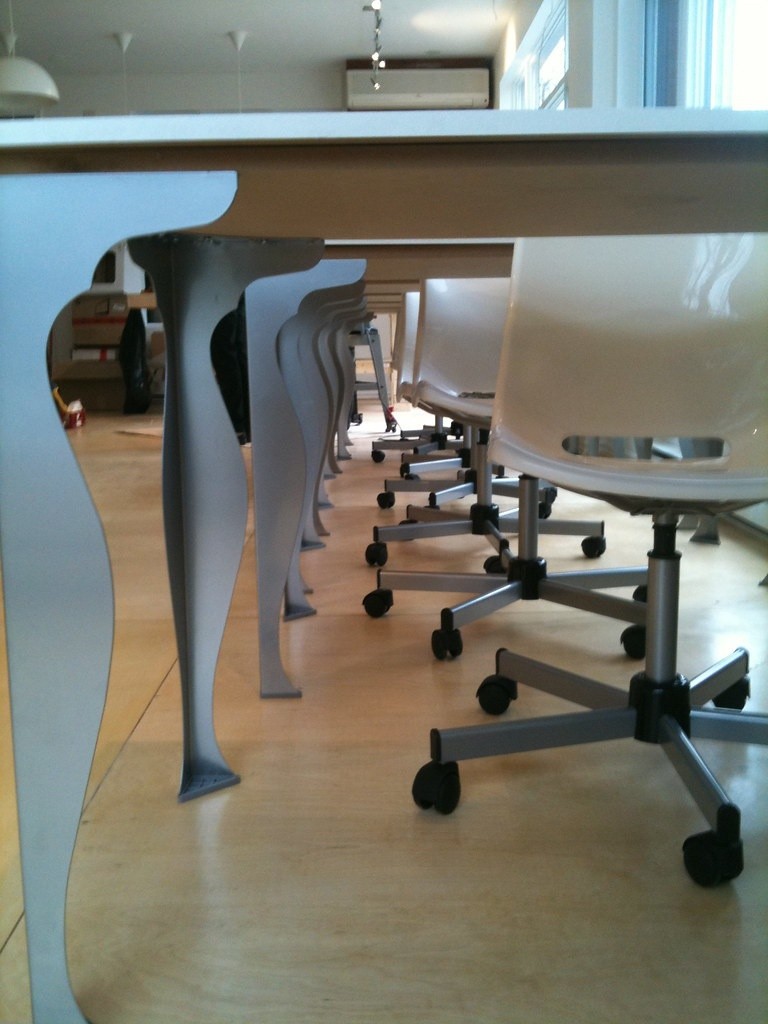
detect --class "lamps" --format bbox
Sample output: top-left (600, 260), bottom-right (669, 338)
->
top-left (1, 35), bottom-right (59, 114)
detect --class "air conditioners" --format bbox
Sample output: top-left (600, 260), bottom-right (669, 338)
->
top-left (346, 67), bottom-right (489, 108)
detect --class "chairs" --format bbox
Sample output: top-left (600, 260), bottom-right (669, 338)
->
top-left (362, 276), bottom-right (650, 661)
top-left (413, 235), bottom-right (768, 887)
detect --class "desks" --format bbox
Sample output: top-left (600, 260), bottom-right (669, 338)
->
top-left (1, 111), bottom-right (768, 1024)
